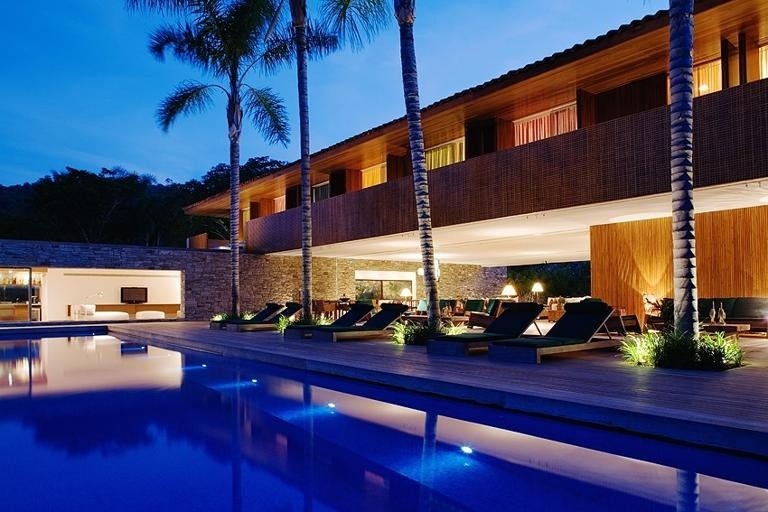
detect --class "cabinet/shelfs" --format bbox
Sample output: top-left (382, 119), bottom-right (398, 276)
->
top-left (67, 303), bottom-right (180, 318)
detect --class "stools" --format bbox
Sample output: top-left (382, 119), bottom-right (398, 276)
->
top-left (136, 311), bottom-right (165, 319)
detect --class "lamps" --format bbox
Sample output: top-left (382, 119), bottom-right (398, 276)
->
top-left (400, 288), bottom-right (412, 305)
top-left (532, 283), bottom-right (543, 304)
top-left (500, 285), bottom-right (517, 299)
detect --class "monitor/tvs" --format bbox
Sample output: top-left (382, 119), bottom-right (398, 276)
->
top-left (121, 341), bottom-right (148, 356)
top-left (121, 287), bottom-right (147, 304)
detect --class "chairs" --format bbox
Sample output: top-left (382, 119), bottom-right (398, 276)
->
top-left (313, 296), bottom-right (568, 324)
top-left (425, 303), bottom-right (545, 357)
top-left (284, 302), bottom-right (374, 339)
top-left (212, 302), bottom-right (304, 332)
top-left (312, 302), bottom-right (410, 343)
top-left (487, 303), bottom-right (624, 364)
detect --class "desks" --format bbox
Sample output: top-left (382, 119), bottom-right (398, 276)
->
top-left (657, 322), bottom-right (750, 340)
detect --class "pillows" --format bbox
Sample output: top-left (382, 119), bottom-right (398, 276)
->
top-left (80, 305), bottom-right (95, 315)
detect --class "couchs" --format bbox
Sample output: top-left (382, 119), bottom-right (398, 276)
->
top-left (647, 297), bottom-right (768, 338)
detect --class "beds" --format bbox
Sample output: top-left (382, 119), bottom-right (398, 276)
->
top-left (71, 304), bottom-right (129, 320)
